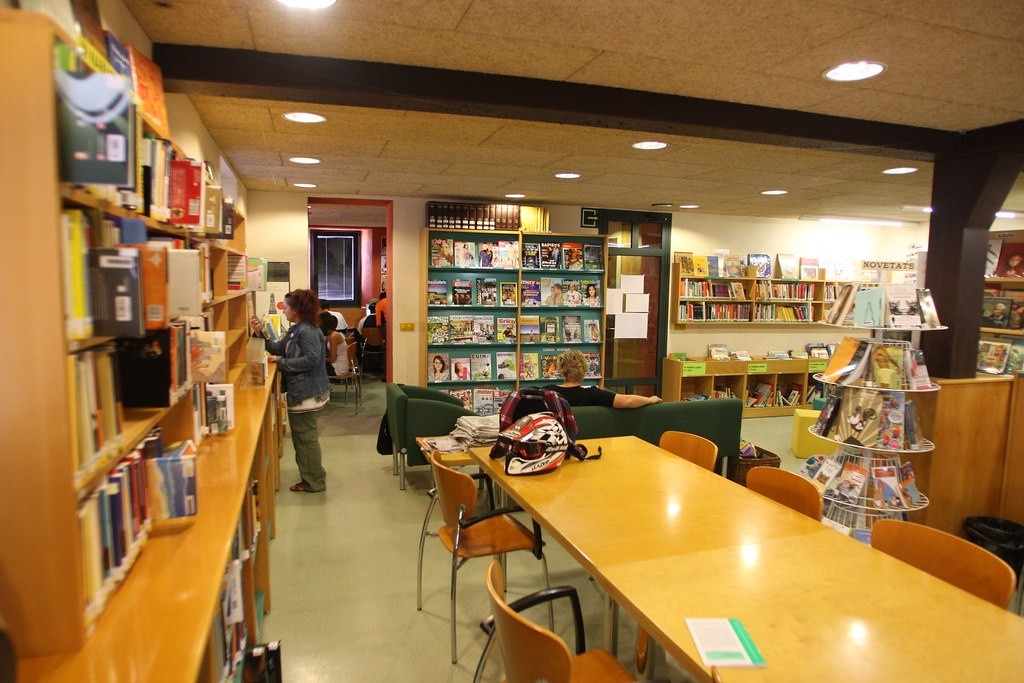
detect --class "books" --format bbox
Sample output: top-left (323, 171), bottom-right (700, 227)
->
top-left (713, 384), bottom-right (738, 400)
top-left (763, 341), bottom-right (835, 361)
top-left (976, 238), bottom-right (1024, 374)
top-left (424, 199), bottom-right (551, 233)
top-left (707, 343), bottom-right (753, 361)
top-left (679, 278), bottom-right (815, 323)
top-left (798, 281), bottom-right (942, 548)
top-left (428, 237), bottom-right (605, 416)
top-left (684, 617), bottom-right (768, 669)
top-left (745, 382), bottom-right (804, 407)
top-left (682, 391), bottom-right (711, 402)
top-left (674, 249), bottom-right (820, 281)
top-left (668, 352), bottom-right (687, 361)
top-left (13, 0), bottom-right (279, 628)
top-left (211, 477), bottom-right (261, 677)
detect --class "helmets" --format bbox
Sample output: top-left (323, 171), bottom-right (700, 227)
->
top-left (498, 412), bottom-right (568, 475)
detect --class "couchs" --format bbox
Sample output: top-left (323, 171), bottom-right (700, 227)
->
top-left (564, 398), bottom-right (742, 480)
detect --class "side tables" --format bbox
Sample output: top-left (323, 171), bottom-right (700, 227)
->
top-left (416, 434), bottom-right (498, 512)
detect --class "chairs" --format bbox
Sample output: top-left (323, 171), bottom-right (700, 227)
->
top-left (386, 383), bottom-right (481, 490)
top-left (416, 451), bottom-right (554, 665)
top-left (315, 328), bottom-right (386, 416)
top-left (473, 559), bottom-right (637, 683)
top-left (870, 519), bottom-right (1017, 610)
top-left (659, 431), bottom-right (719, 472)
top-left (746, 466), bottom-right (823, 523)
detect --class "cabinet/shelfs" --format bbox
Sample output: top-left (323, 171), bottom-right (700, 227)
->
top-left (418, 228), bottom-right (522, 416)
top-left (0, 8), bottom-right (285, 683)
top-left (519, 229), bottom-right (609, 389)
top-left (902, 215), bottom-right (1024, 539)
top-left (662, 343), bottom-right (838, 419)
top-left (671, 249), bottom-right (917, 324)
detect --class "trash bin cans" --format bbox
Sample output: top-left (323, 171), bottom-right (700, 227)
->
top-left (967, 516), bottom-right (1024, 589)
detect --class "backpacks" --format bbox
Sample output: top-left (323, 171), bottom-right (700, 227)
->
top-left (489, 385), bottom-right (602, 462)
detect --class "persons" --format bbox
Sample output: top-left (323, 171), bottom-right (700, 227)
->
top-left (589, 324), bottom-right (599, 341)
top-left (454, 362), bottom-right (470, 380)
top-left (564, 282), bottom-right (581, 305)
top-left (466, 391), bottom-right (470, 398)
top-left (553, 283), bottom-right (562, 304)
top-left (541, 350), bottom-right (664, 409)
top-left (478, 242), bottom-right (494, 267)
top-left (318, 299), bottom-right (348, 330)
top-left (501, 324), bottom-right (516, 339)
top-left (375, 291), bottom-right (386, 327)
top-left (357, 302), bottom-right (385, 335)
top-left (582, 284), bottom-right (601, 299)
top-left (461, 392), bottom-right (468, 401)
top-left (529, 329), bottom-right (533, 342)
top-left (319, 312), bottom-right (350, 375)
top-left (430, 354), bottom-right (449, 381)
top-left (456, 393), bottom-right (461, 399)
top-left (545, 286), bottom-right (555, 305)
top-left (250, 288), bottom-right (332, 492)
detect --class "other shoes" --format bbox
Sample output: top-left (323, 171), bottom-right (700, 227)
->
top-left (290, 482), bottom-right (308, 491)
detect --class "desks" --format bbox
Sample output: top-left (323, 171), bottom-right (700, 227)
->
top-left (467, 435), bottom-right (1024, 683)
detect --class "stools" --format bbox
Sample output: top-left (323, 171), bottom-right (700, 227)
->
top-left (813, 398), bottom-right (828, 411)
top-left (792, 409), bottom-right (840, 459)
